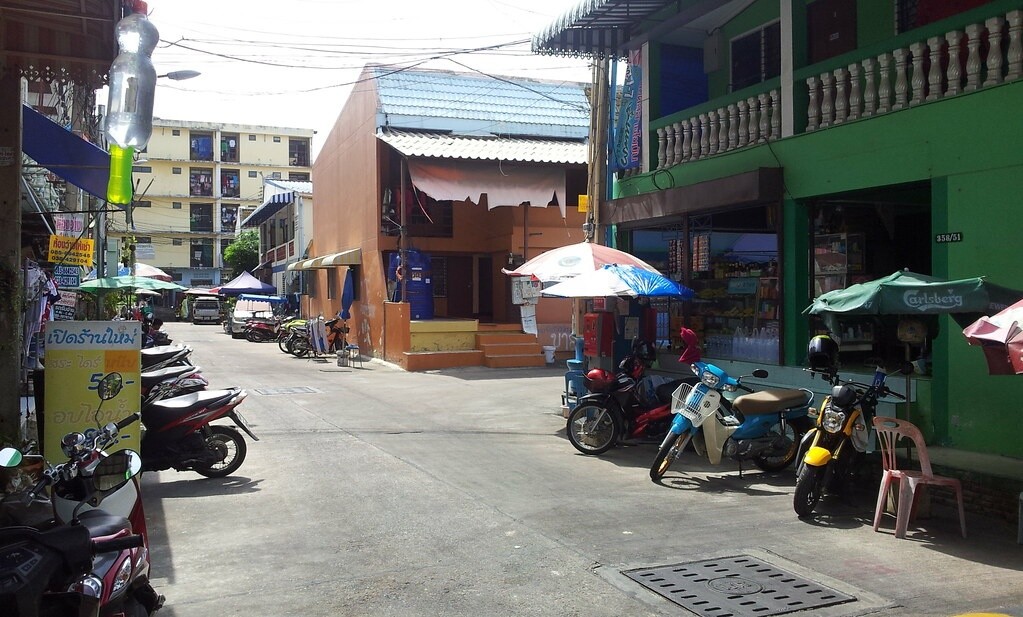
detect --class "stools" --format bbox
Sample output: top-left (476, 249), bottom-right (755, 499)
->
top-left (345, 344), bottom-right (362, 368)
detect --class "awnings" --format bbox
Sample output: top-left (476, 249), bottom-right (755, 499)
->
top-left (21, 100), bottom-right (132, 217)
top-left (179, 287), bottom-right (224, 296)
top-left (240, 189), bottom-right (295, 229)
top-left (286, 245), bottom-right (361, 270)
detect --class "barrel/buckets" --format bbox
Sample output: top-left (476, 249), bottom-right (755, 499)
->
top-left (543, 346), bottom-right (556, 363)
top-left (336, 349), bottom-right (349, 366)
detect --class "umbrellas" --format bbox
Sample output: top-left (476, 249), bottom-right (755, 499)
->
top-left (502, 238), bottom-right (663, 361)
top-left (339, 267), bottom-right (354, 350)
top-left (77, 276), bottom-right (188, 322)
top-left (539, 264), bottom-right (696, 328)
top-left (217, 270), bottom-right (277, 299)
top-left (963, 299), bottom-right (1023, 376)
top-left (801, 266), bottom-right (1017, 468)
top-left (83, 259), bottom-right (174, 281)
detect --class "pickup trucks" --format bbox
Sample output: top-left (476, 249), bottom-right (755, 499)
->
top-left (193, 297), bottom-right (221, 324)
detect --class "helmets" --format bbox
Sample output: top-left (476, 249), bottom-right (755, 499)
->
top-left (808, 335), bottom-right (841, 374)
top-left (588, 369), bottom-right (615, 388)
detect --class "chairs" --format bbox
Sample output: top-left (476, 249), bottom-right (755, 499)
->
top-left (872, 416), bottom-right (967, 538)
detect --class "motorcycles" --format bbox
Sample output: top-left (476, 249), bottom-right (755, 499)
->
top-left (566, 335), bottom-right (735, 456)
top-left (791, 365), bottom-right (908, 518)
top-left (244, 306), bottom-right (352, 358)
top-left (141, 316), bottom-right (259, 479)
top-left (0, 370), bottom-right (165, 617)
top-left (649, 329), bottom-right (815, 483)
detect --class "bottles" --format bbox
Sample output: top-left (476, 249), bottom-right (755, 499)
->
top-left (713, 263), bottom-right (753, 278)
top-left (105, 0), bottom-right (159, 148)
top-left (107, 143), bottom-right (133, 205)
top-left (706, 335), bottom-right (733, 360)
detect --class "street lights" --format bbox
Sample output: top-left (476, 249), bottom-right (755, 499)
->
top-left (95, 69), bottom-right (204, 320)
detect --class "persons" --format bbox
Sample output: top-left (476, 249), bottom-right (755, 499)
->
top-left (113, 296), bottom-right (149, 321)
top-left (181, 294), bottom-right (190, 321)
top-left (149, 319), bottom-right (171, 345)
